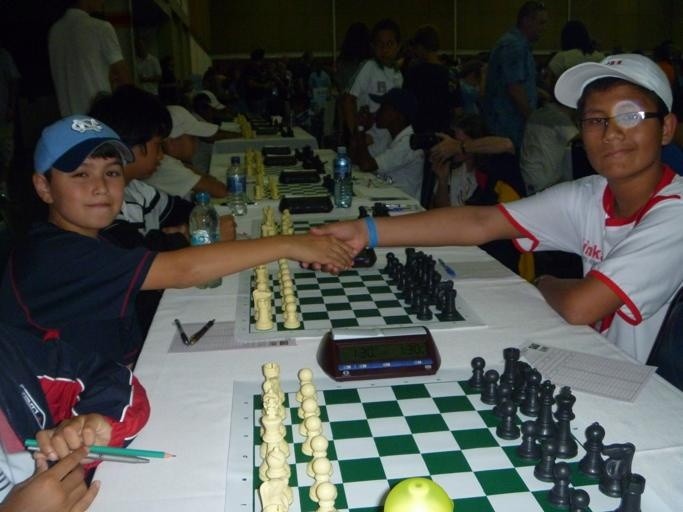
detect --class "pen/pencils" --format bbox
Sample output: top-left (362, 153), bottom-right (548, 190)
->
top-left (174, 319), bottom-right (189, 345)
top-left (190, 319), bottom-right (215, 345)
top-left (24, 439), bottom-right (176, 464)
top-left (438, 258), bottom-right (456, 276)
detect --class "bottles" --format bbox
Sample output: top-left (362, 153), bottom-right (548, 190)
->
top-left (332, 146), bottom-right (353, 209)
top-left (225, 155), bottom-right (247, 218)
top-left (187, 192), bottom-right (222, 289)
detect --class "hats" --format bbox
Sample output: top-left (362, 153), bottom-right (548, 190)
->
top-left (157, 105), bottom-right (218, 138)
top-left (193, 90), bottom-right (225, 109)
top-left (555, 53), bottom-right (673, 114)
top-left (369, 87), bottom-right (404, 110)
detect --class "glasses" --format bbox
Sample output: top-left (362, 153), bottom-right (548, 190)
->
top-left (35, 115), bottom-right (136, 174)
top-left (574, 110), bottom-right (667, 132)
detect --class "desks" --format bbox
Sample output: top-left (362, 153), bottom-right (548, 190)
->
top-left (84, 115), bottom-right (681, 512)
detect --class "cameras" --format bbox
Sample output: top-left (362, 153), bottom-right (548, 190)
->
top-left (408, 122), bottom-right (455, 150)
